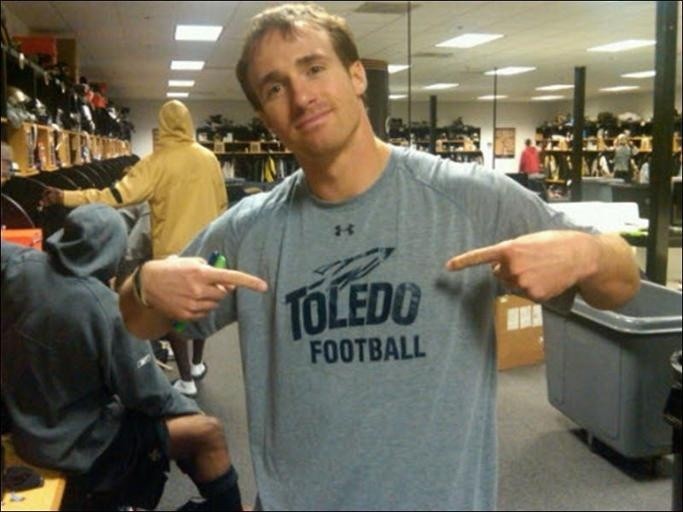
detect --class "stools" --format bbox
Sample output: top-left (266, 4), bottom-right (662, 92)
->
top-left (0, 431), bottom-right (70, 511)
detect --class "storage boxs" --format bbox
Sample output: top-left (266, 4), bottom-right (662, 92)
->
top-left (496, 295), bottom-right (544, 370)
top-left (13, 36), bottom-right (80, 86)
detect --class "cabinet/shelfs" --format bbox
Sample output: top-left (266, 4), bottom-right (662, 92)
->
top-left (388, 126), bottom-right (481, 164)
top-left (0, 43), bottom-right (142, 254)
top-left (534, 124), bottom-right (683, 185)
top-left (194, 127), bottom-right (300, 182)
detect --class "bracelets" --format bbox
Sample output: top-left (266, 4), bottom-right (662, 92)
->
top-left (130, 261), bottom-right (153, 309)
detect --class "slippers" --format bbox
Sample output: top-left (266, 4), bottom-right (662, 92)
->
top-left (171, 362), bottom-right (207, 398)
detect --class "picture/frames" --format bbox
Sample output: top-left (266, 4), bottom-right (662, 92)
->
top-left (495, 127), bottom-right (515, 158)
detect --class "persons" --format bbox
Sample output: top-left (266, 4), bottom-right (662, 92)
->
top-left (608, 133), bottom-right (633, 184)
top-left (36, 98), bottom-right (235, 397)
top-left (107, 0), bottom-right (639, 510)
top-left (0, 200), bottom-right (250, 512)
top-left (517, 137), bottom-right (540, 174)
